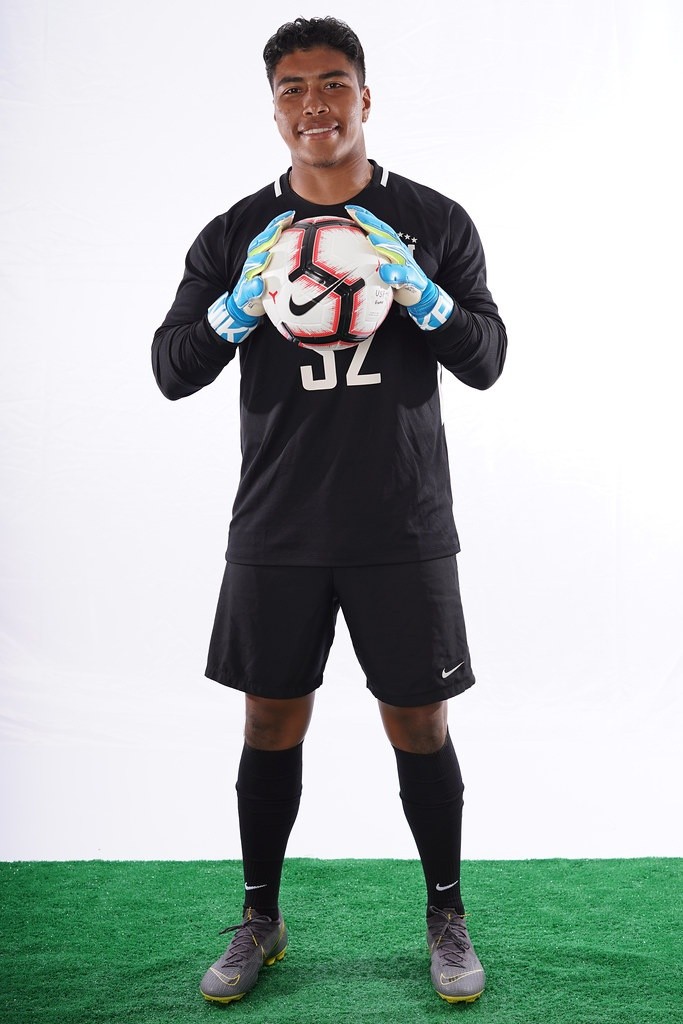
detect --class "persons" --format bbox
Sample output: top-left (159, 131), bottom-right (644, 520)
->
top-left (153, 15), bottom-right (510, 1004)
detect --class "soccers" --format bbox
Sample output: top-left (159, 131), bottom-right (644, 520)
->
top-left (259, 215), bottom-right (394, 352)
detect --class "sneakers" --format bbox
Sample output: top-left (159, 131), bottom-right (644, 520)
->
top-left (426, 907), bottom-right (487, 1003)
top-left (201, 908), bottom-right (289, 1003)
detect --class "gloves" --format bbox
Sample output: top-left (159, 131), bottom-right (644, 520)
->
top-left (345, 202), bottom-right (454, 331)
top-left (207, 208), bottom-right (296, 344)
